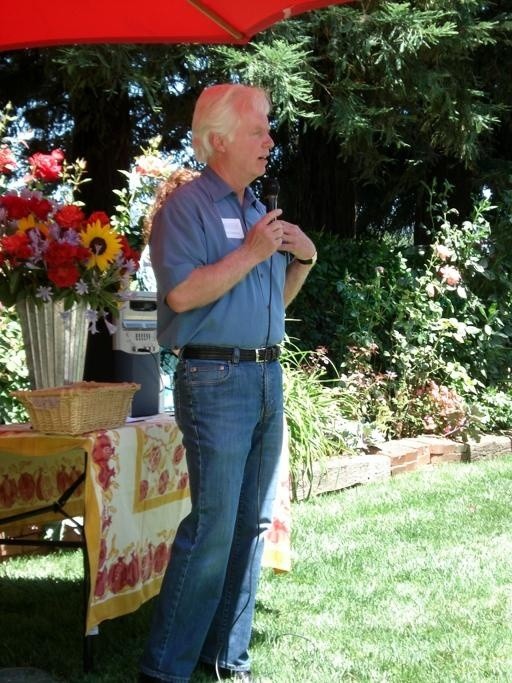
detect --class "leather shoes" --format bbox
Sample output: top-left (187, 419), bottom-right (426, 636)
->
top-left (204, 666), bottom-right (251, 679)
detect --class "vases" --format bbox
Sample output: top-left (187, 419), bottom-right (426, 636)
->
top-left (15, 288), bottom-right (92, 393)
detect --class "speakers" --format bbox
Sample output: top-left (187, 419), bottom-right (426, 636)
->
top-left (85, 292), bottom-right (161, 418)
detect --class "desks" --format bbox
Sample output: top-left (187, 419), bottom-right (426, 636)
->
top-left (0, 411), bottom-right (290, 675)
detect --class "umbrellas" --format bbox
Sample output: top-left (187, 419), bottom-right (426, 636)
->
top-left (0, 0), bottom-right (350, 50)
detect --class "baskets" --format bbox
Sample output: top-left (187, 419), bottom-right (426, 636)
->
top-left (9, 383), bottom-right (141, 435)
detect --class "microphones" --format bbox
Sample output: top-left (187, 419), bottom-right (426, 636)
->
top-left (264, 180), bottom-right (280, 221)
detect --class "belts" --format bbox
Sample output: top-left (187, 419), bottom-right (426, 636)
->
top-left (183, 346), bottom-right (280, 361)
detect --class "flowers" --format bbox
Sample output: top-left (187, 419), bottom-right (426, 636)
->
top-left (0, 100), bottom-right (166, 337)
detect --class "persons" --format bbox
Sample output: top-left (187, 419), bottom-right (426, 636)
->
top-left (130, 85), bottom-right (318, 680)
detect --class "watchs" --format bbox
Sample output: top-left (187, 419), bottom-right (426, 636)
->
top-left (295, 250), bottom-right (318, 264)
